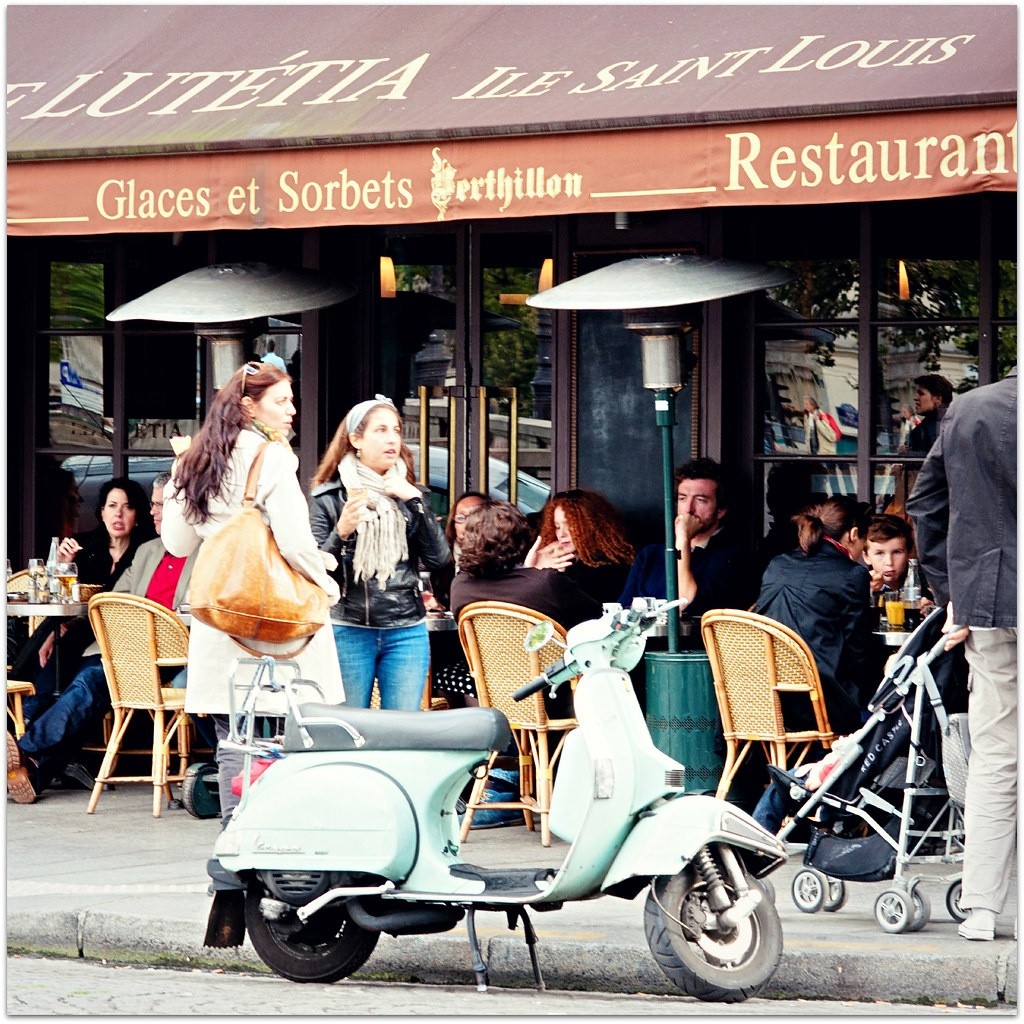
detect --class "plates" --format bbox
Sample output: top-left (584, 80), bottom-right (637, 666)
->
top-left (7, 592), bottom-right (29, 601)
top-left (424, 611), bottom-right (445, 619)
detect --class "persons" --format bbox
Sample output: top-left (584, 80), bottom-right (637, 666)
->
top-left (7, 472), bottom-right (203, 804)
top-left (160, 359), bottom-right (347, 818)
top-left (802, 396), bottom-right (847, 498)
top-left (883, 373), bottom-right (954, 516)
top-left (428, 456), bottom-right (761, 832)
top-left (307, 395), bottom-right (455, 711)
top-left (906, 366), bottom-right (1017, 942)
top-left (751, 494), bottom-right (967, 839)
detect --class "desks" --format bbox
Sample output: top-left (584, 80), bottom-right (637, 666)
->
top-left (7, 602), bottom-right (115, 792)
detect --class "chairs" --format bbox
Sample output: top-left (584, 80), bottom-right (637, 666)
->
top-left (701, 609), bottom-right (848, 804)
top-left (457, 601), bottom-right (580, 848)
top-left (86, 591), bottom-right (206, 818)
top-left (7, 680), bottom-right (36, 742)
top-left (7, 568), bottom-right (80, 640)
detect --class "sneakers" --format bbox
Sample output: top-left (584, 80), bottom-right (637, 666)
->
top-left (457, 791), bottom-right (526, 829)
top-left (487, 767), bottom-right (520, 789)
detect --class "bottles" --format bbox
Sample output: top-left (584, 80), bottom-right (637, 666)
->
top-left (46, 536), bottom-right (60, 593)
top-left (419, 571), bottom-right (433, 578)
top-left (903, 559), bottom-right (922, 631)
top-left (38, 587), bottom-right (50, 603)
top-left (7, 559), bottom-right (12, 580)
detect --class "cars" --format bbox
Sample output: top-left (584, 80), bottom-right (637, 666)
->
top-left (404, 442), bottom-right (553, 524)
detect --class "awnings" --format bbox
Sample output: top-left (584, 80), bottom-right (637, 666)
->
top-left (6, 4), bottom-right (1017, 235)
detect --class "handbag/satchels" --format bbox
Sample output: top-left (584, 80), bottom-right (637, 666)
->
top-left (808, 418), bottom-right (820, 455)
top-left (917, 651), bottom-right (972, 812)
top-left (822, 412), bottom-right (843, 442)
top-left (187, 441), bottom-right (330, 644)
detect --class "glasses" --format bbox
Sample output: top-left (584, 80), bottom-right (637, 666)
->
top-left (239, 359), bottom-right (261, 394)
top-left (453, 513), bottom-right (473, 522)
top-left (150, 500), bottom-right (163, 508)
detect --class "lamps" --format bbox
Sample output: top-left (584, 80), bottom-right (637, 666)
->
top-left (614, 212), bottom-right (628, 229)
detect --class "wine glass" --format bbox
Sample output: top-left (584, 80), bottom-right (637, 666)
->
top-left (54, 562), bottom-right (79, 603)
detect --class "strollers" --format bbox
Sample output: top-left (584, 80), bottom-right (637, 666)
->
top-left (763, 615), bottom-right (972, 934)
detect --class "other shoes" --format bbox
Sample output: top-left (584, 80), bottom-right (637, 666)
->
top-left (7, 730), bottom-right (36, 804)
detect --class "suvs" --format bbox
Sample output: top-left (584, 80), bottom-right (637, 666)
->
top-left (61, 455), bottom-right (183, 519)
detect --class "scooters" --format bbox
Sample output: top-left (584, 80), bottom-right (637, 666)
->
top-left (200, 593), bottom-right (786, 1003)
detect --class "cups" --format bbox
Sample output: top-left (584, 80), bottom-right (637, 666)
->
top-left (31, 565), bottom-right (52, 587)
top-left (870, 588), bottom-right (937, 632)
top-left (28, 558), bottom-right (45, 604)
top-left (418, 578), bottom-right (434, 608)
